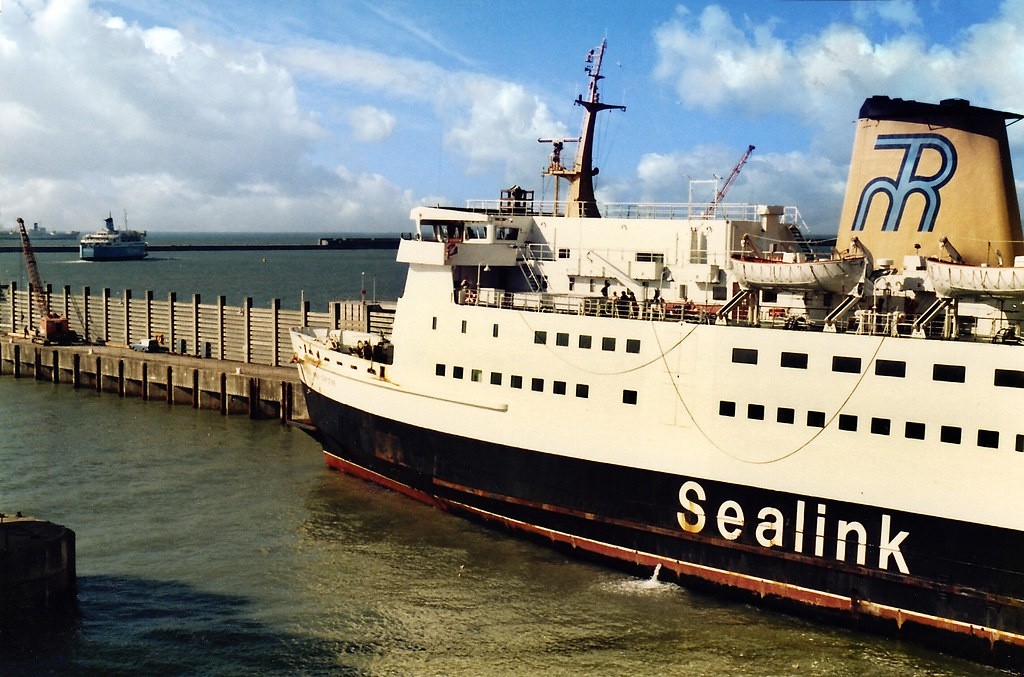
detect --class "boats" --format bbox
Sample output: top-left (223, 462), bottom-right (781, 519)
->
top-left (78, 212), bottom-right (149, 260)
top-left (289, 36), bottom-right (1024, 664)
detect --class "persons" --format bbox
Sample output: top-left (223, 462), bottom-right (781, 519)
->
top-left (24, 325), bottom-right (29, 338)
top-left (461, 279), bottom-right (477, 293)
top-left (609, 291), bottom-right (639, 320)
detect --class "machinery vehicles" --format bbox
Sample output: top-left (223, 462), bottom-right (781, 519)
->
top-left (17, 218), bottom-right (76, 345)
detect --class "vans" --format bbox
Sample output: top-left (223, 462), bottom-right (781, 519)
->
top-left (131, 340), bottom-right (159, 353)
